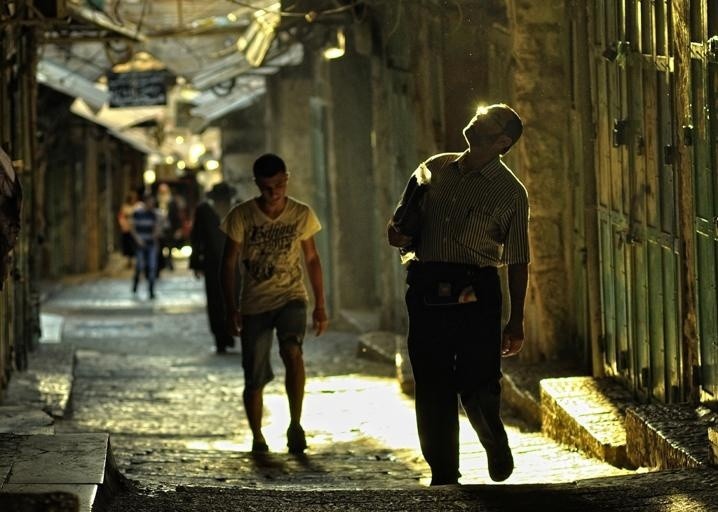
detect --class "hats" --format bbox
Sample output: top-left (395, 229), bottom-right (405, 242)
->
top-left (206, 180), bottom-right (236, 201)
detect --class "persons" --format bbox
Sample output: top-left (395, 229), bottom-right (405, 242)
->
top-left (219, 152), bottom-right (329, 452)
top-left (386, 101), bottom-right (531, 486)
top-left (207, 180), bottom-right (242, 338)
top-left (189, 200), bottom-right (242, 356)
top-left (116, 182), bottom-right (185, 301)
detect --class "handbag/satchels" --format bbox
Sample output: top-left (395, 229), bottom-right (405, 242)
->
top-left (390, 162), bottom-right (433, 256)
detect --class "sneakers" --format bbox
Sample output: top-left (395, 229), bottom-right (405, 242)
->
top-left (287, 426), bottom-right (308, 453)
top-left (489, 436), bottom-right (513, 480)
